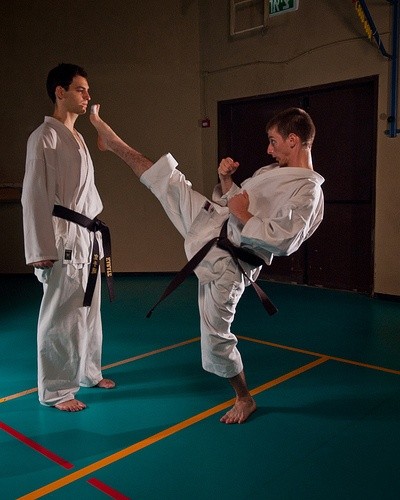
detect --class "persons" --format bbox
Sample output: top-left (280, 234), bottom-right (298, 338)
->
top-left (88, 104), bottom-right (325, 424)
top-left (20, 64), bottom-right (116, 411)
